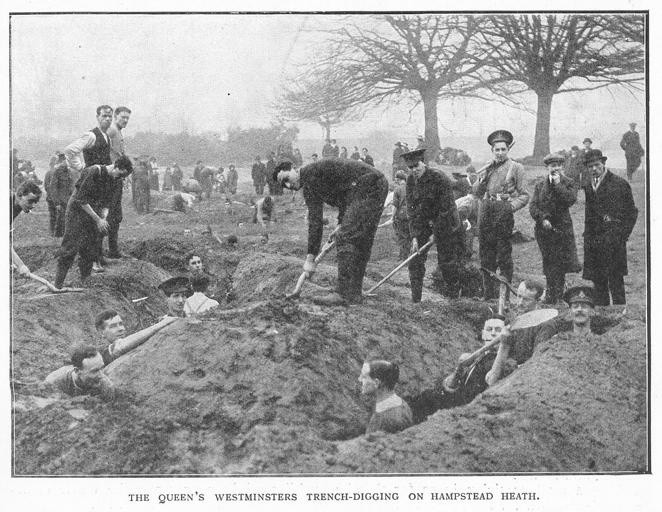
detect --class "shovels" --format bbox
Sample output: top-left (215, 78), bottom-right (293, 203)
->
top-left (285, 240), bottom-right (338, 301)
top-left (461, 308), bottom-right (558, 364)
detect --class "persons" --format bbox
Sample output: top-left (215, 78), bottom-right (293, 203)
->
top-left (51, 155), bottom-right (135, 287)
top-left (156, 274), bottom-right (193, 319)
top-left (472, 128), bottom-right (530, 295)
top-left (183, 272), bottom-right (219, 314)
top-left (356, 359), bottom-right (414, 439)
top-left (11, 135), bottom-right (645, 231)
top-left (181, 250), bottom-right (210, 272)
top-left (620, 120), bottom-right (644, 179)
top-left (405, 147), bottom-right (482, 302)
top-left (105, 106), bottom-right (133, 259)
top-left (269, 158), bottom-right (393, 308)
top-left (579, 151), bottom-right (639, 304)
top-left (561, 273), bottom-right (603, 335)
top-left (63, 105), bottom-right (112, 270)
top-left (95, 307), bottom-right (181, 366)
top-left (511, 274), bottom-right (547, 317)
top-left (439, 312), bottom-right (517, 400)
top-left (530, 152), bottom-right (585, 305)
top-left (45, 345), bottom-right (113, 389)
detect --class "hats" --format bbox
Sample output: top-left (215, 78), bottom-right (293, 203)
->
top-left (401, 149), bottom-right (428, 164)
top-left (393, 135), bottom-right (425, 147)
top-left (159, 277), bottom-right (190, 292)
top-left (571, 139), bottom-right (608, 163)
top-left (544, 152), bottom-right (565, 164)
top-left (488, 127), bottom-right (514, 146)
top-left (563, 285), bottom-right (600, 310)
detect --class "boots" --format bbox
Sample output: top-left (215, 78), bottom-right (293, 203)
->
top-left (313, 243), bottom-right (370, 306)
top-left (79, 256), bottom-right (102, 287)
top-left (55, 257), bottom-right (71, 290)
